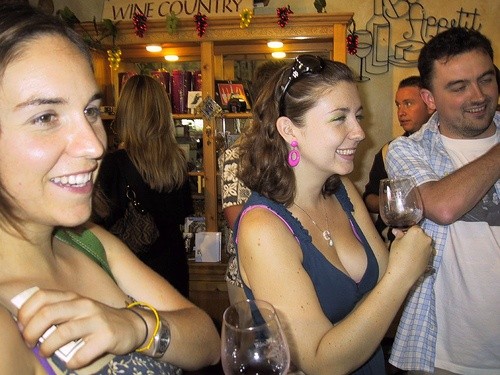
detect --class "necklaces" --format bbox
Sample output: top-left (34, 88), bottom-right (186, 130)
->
top-left (292, 199), bottom-right (334, 249)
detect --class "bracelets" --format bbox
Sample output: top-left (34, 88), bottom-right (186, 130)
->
top-left (123, 301), bottom-right (159, 356)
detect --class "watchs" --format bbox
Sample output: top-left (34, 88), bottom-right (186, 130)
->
top-left (146, 308), bottom-right (171, 360)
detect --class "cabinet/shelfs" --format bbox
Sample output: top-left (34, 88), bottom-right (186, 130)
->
top-left (93, 12), bottom-right (354, 230)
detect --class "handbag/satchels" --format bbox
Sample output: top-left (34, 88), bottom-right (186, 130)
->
top-left (107, 150), bottom-right (161, 257)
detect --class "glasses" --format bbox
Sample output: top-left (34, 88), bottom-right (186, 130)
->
top-left (278, 55), bottom-right (325, 117)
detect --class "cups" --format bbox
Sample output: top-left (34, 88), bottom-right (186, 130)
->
top-left (222, 299), bottom-right (291, 375)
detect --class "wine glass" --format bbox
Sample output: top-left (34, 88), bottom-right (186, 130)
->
top-left (379, 176), bottom-right (425, 239)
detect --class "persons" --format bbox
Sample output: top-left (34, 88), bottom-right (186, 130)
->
top-left (221, 61), bottom-right (288, 353)
top-left (236, 55), bottom-right (431, 375)
top-left (388, 26), bottom-right (500, 375)
top-left (89, 73), bottom-right (194, 298)
top-left (0, 0), bottom-right (219, 375)
top-left (362, 75), bottom-right (431, 253)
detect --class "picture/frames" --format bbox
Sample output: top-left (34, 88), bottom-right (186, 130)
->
top-left (215, 79), bottom-right (253, 111)
